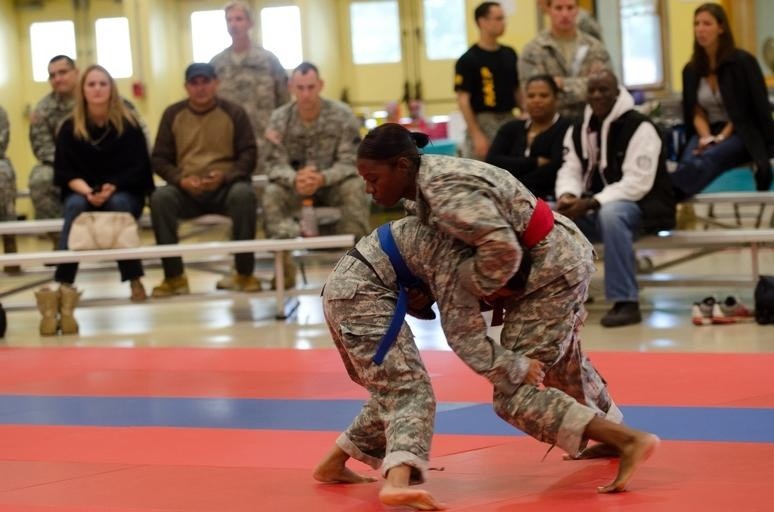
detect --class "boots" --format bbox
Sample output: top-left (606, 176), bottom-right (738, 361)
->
top-left (271, 252), bottom-right (295, 289)
top-left (59, 286), bottom-right (82, 336)
top-left (34, 288), bottom-right (61, 336)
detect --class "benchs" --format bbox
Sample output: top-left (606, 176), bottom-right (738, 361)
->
top-left (0, 171), bottom-right (351, 324)
top-left (589, 157), bottom-right (774, 300)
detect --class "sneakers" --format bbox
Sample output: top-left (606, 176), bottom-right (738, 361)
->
top-left (600, 301), bottom-right (641, 326)
top-left (712, 296), bottom-right (756, 323)
top-left (153, 277), bottom-right (188, 296)
top-left (692, 297), bottom-right (715, 324)
top-left (235, 274), bottom-right (259, 290)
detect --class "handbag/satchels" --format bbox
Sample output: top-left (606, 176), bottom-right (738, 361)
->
top-left (67, 210), bottom-right (140, 251)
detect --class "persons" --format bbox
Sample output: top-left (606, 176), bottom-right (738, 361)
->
top-left (1, 3), bottom-right (373, 304)
top-left (313, 216), bottom-right (546, 510)
top-left (356, 122), bottom-right (660, 494)
top-left (451, 3), bottom-right (773, 328)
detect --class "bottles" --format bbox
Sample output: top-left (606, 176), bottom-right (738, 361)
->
top-left (299, 200), bottom-right (318, 237)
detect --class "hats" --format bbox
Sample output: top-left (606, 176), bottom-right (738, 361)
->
top-left (186, 63), bottom-right (213, 80)
